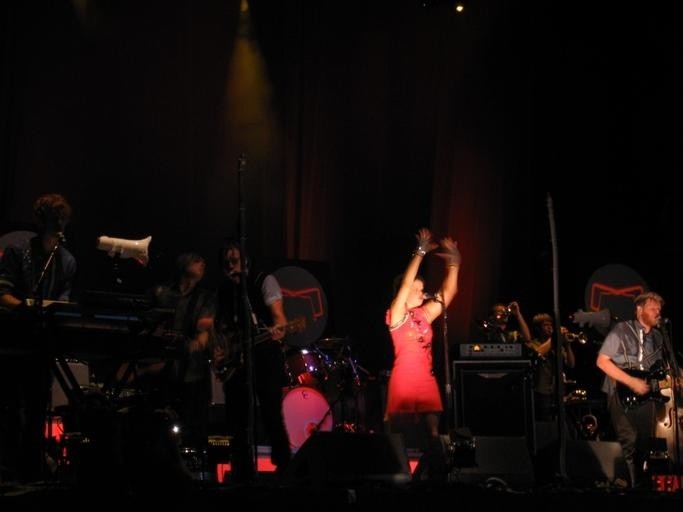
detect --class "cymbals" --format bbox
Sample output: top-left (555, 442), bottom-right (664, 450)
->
top-left (313, 338), bottom-right (350, 343)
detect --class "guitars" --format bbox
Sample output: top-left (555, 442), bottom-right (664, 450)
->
top-left (205, 316), bottom-right (300, 382)
top-left (616, 366), bottom-right (683, 409)
top-left (325, 358), bottom-right (362, 397)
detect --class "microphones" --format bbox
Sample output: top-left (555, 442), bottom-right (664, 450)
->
top-left (421, 293), bottom-right (436, 301)
top-left (55, 230), bottom-right (67, 243)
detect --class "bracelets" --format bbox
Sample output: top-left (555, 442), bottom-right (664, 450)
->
top-left (447, 259), bottom-right (459, 268)
top-left (412, 245), bottom-right (426, 257)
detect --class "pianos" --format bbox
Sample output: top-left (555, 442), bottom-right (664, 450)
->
top-left (0, 291), bottom-right (177, 330)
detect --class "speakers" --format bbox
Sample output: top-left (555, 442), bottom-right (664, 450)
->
top-left (284, 428), bottom-right (411, 485)
top-left (472, 433), bottom-right (536, 479)
top-left (568, 441), bottom-right (625, 484)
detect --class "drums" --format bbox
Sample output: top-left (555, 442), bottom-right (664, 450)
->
top-left (282, 386), bottom-right (333, 454)
top-left (284, 350), bottom-right (329, 389)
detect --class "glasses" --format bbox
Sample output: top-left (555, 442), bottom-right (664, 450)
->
top-left (223, 257), bottom-right (239, 265)
top-left (183, 272), bottom-right (201, 281)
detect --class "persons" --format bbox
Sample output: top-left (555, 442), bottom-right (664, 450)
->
top-left (465, 300), bottom-right (532, 434)
top-left (210, 238), bottom-right (289, 489)
top-left (129, 248), bottom-right (216, 450)
top-left (382, 224), bottom-right (464, 440)
top-left (527, 312), bottom-right (578, 422)
top-left (593, 291), bottom-right (665, 487)
top-left (1, 191), bottom-right (80, 483)
